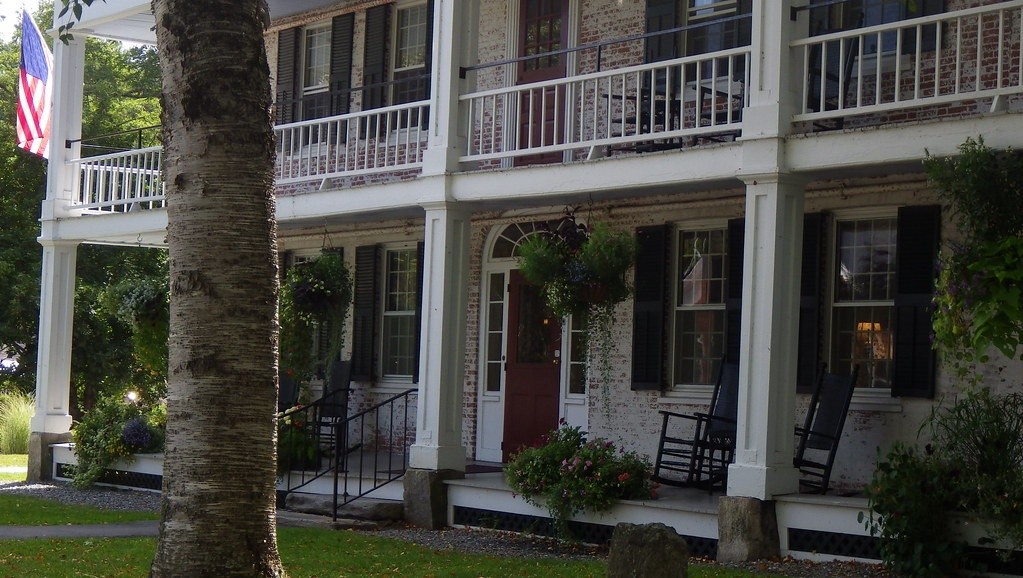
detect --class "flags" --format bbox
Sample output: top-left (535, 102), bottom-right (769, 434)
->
top-left (16, 7), bottom-right (52, 159)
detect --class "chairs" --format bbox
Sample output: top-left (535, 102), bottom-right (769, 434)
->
top-left (642, 349), bottom-right (741, 497)
top-left (597, 42), bottom-right (687, 156)
top-left (791, 358), bottom-right (862, 496)
top-left (806, 12), bottom-right (865, 134)
top-left (692, 32), bottom-right (752, 146)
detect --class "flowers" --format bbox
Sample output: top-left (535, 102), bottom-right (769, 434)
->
top-left (541, 277), bottom-right (576, 327)
top-left (276, 404), bottom-right (309, 430)
top-left (929, 242), bottom-right (982, 364)
top-left (503, 417), bottom-right (663, 533)
top-left (279, 270), bottom-right (333, 329)
